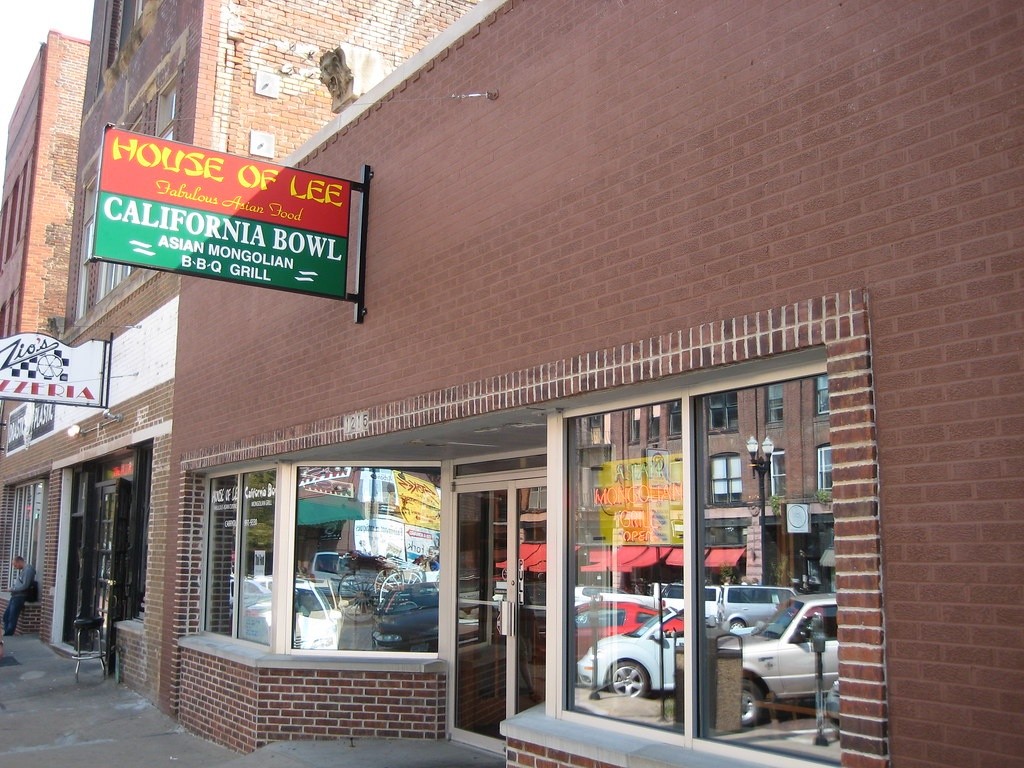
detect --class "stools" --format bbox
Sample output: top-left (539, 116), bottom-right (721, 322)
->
top-left (72, 617), bottom-right (107, 683)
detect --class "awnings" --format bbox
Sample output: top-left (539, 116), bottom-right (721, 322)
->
top-left (496, 542), bottom-right (746, 572)
top-left (297, 496), bottom-right (366, 525)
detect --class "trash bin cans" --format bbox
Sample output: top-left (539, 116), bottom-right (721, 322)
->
top-left (673, 628), bottom-right (745, 735)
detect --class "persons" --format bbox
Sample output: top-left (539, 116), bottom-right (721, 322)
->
top-left (3, 556), bottom-right (35, 636)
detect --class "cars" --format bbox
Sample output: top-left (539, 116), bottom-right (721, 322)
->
top-left (239, 551), bottom-right (439, 655)
top-left (457, 577), bottom-right (840, 727)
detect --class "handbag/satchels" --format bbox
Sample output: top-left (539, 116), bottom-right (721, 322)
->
top-left (26, 581), bottom-right (38, 602)
